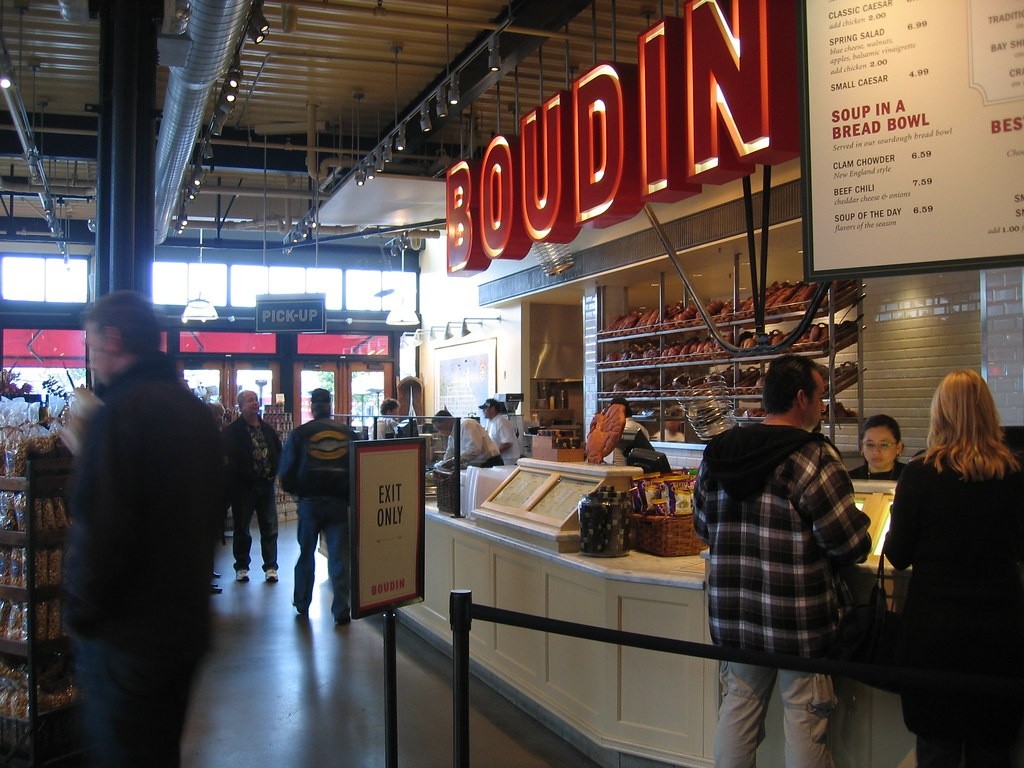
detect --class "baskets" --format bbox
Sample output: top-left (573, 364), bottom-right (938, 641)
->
top-left (632, 510), bottom-right (708, 556)
top-left (432, 468), bottom-right (455, 515)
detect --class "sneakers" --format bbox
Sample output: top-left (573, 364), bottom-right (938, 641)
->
top-left (265, 569), bottom-right (279, 582)
top-left (236, 570), bottom-right (250, 582)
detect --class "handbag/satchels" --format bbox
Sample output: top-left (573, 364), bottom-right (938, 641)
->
top-left (842, 551), bottom-right (914, 692)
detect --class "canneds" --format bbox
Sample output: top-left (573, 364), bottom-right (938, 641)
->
top-left (262, 405), bottom-right (293, 440)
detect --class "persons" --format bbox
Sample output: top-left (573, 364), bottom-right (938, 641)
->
top-left (885, 369), bottom-right (1024, 768)
top-left (690, 353), bottom-right (872, 768)
top-left (206, 402), bottom-right (224, 428)
top-left (431, 410), bottom-right (504, 469)
top-left (848, 414), bottom-right (907, 482)
top-left (607, 398), bottom-right (650, 466)
top-left (277, 389), bottom-right (359, 624)
top-left (374, 398), bottom-right (401, 439)
top-left (223, 392), bottom-right (282, 583)
top-left (479, 399), bottom-right (522, 465)
top-left (653, 419), bottom-right (686, 442)
top-left (57, 290), bottom-right (220, 768)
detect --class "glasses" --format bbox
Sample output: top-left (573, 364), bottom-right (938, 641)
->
top-left (861, 438), bottom-right (898, 451)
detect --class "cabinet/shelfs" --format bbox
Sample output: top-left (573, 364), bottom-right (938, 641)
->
top-left (598, 274), bottom-right (870, 471)
top-left (0, 447), bottom-right (85, 768)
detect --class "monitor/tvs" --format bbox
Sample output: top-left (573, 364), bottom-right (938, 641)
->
top-left (616, 428), bottom-right (655, 457)
top-left (628, 448), bottom-right (672, 474)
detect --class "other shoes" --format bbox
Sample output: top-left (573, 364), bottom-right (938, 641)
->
top-left (212, 582), bottom-right (222, 594)
top-left (213, 571), bottom-right (222, 578)
top-left (334, 613), bottom-right (351, 625)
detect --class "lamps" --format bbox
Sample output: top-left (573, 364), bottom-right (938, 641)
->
top-left (176, 10), bottom-right (279, 323)
top-left (281, 38), bottom-right (508, 257)
top-left (0, 76), bottom-right (71, 269)
top-left (429, 325), bottom-right (461, 340)
top-left (413, 328), bottom-right (447, 347)
top-left (444, 321), bottom-right (484, 339)
top-left (461, 314), bottom-right (501, 339)
top-left (399, 330), bottom-right (428, 348)
top-left (384, 242), bottom-right (419, 327)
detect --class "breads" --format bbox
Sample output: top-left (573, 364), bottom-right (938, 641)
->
top-left (585, 403), bottom-right (626, 463)
top-left (602, 279), bottom-right (862, 419)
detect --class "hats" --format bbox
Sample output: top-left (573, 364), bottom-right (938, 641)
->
top-left (479, 399), bottom-right (498, 409)
